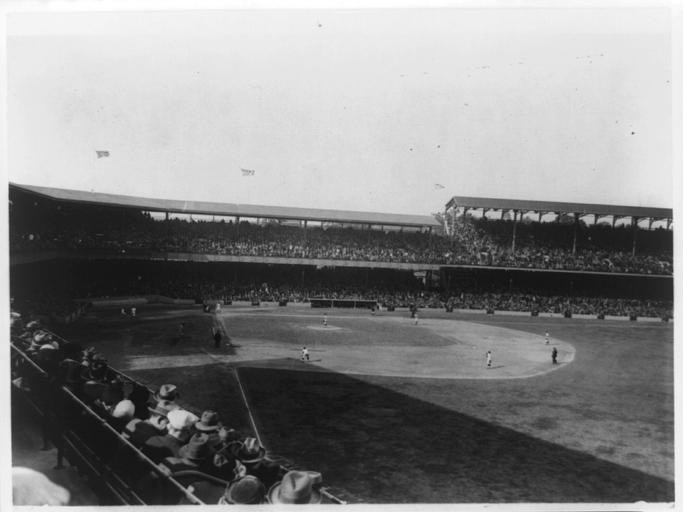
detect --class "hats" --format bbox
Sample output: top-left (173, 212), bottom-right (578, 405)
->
top-left (178, 433), bottom-right (216, 461)
top-left (266, 470), bottom-right (322, 504)
top-left (224, 475), bottom-right (266, 504)
top-left (153, 383), bottom-right (179, 405)
top-left (201, 454), bottom-right (246, 482)
top-left (27, 329), bottom-right (51, 352)
top-left (165, 408), bottom-right (201, 431)
top-left (237, 436), bottom-right (266, 464)
top-left (195, 409), bottom-right (222, 433)
